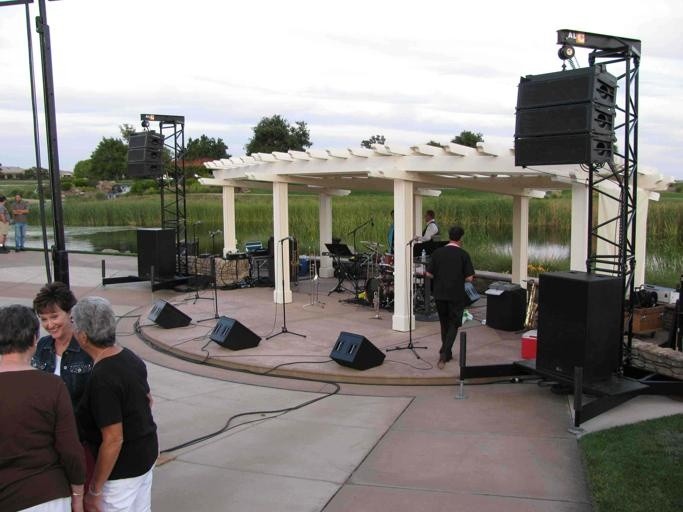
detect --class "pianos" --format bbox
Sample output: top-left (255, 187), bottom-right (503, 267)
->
top-left (226, 249), bottom-right (266, 261)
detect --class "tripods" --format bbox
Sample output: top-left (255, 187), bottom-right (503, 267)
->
top-left (266, 240), bottom-right (383, 340)
top-left (197, 234), bottom-right (220, 322)
top-left (386, 241), bottom-right (425, 359)
top-left (185, 224), bottom-right (214, 304)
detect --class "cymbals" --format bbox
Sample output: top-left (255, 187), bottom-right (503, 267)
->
top-left (360, 241), bottom-right (384, 247)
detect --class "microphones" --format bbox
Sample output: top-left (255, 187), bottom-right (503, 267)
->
top-left (210, 230), bottom-right (221, 238)
top-left (278, 237), bottom-right (289, 242)
top-left (192, 222), bottom-right (203, 225)
top-left (407, 237), bottom-right (419, 245)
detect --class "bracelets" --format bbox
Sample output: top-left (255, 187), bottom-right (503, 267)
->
top-left (69, 488), bottom-right (85, 497)
top-left (87, 488), bottom-right (102, 498)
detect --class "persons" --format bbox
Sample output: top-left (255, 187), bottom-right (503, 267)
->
top-left (25, 279), bottom-right (96, 512)
top-left (385, 209), bottom-right (395, 254)
top-left (424, 225), bottom-right (476, 370)
top-left (0, 194), bottom-right (10, 254)
top-left (0, 303), bottom-right (87, 511)
top-left (657, 279), bottom-right (682, 353)
top-left (421, 208), bottom-right (440, 243)
top-left (68, 295), bottom-right (160, 512)
top-left (11, 193), bottom-right (29, 253)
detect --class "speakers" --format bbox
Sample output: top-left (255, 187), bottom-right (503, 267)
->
top-left (534, 270), bottom-right (624, 385)
top-left (137, 228), bottom-right (176, 277)
top-left (331, 332), bottom-right (385, 370)
top-left (514, 65), bottom-right (617, 168)
top-left (209, 316), bottom-right (262, 350)
top-left (127, 133), bottom-right (164, 177)
top-left (486, 289), bottom-right (526, 332)
top-left (147, 299), bottom-right (192, 329)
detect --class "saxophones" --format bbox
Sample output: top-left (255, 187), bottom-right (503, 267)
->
top-left (522, 279), bottom-right (539, 329)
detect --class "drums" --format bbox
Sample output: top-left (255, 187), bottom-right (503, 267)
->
top-left (412, 261), bottom-right (427, 278)
top-left (379, 252), bottom-right (394, 273)
top-left (413, 277), bottom-right (424, 289)
top-left (365, 273), bottom-right (394, 308)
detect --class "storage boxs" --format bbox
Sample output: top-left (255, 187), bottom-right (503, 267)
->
top-left (520, 329), bottom-right (537, 361)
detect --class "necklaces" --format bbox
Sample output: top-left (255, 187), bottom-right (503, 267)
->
top-left (89, 347), bottom-right (110, 372)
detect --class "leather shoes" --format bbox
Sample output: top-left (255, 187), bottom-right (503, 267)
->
top-left (16, 249), bottom-right (27, 252)
top-left (439, 348), bottom-right (452, 362)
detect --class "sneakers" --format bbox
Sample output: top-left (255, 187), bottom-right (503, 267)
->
top-left (0, 247), bottom-right (10, 253)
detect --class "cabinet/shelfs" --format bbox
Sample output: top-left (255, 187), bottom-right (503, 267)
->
top-left (624, 305), bottom-right (665, 340)
